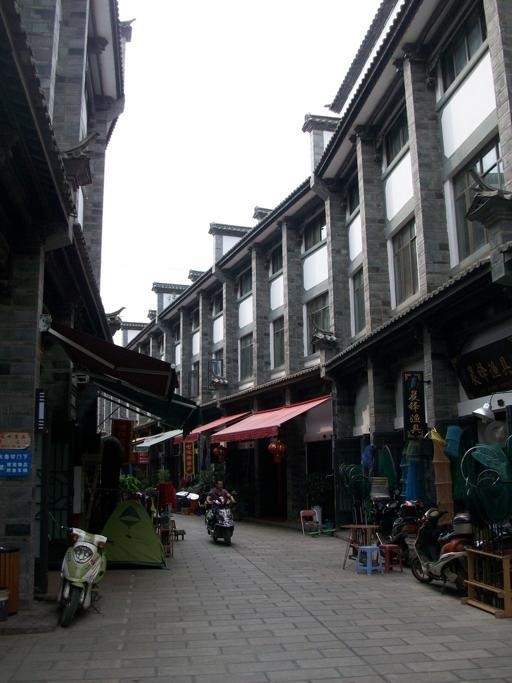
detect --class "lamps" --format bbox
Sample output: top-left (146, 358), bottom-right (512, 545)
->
top-left (36, 388), bottom-right (50, 437)
top-left (471, 389), bottom-right (511, 423)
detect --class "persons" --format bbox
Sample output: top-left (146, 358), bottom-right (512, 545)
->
top-left (156, 475), bottom-right (191, 512)
top-left (205, 480), bottom-right (236, 528)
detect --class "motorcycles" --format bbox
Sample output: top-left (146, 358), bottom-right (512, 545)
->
top-left (58, 524), bottom-right (114, 629)
top-left (411, 506), bottom-right (479, 596)
top-left (369, 488), bottom-right (421, 567)
top-left (203, 490), bottom-right (238, 546)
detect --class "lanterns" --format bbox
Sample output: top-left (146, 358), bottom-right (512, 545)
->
top-left (267, 440), bottom-right (286, 464)
top-left (213, 445), bottom-right (228, 464)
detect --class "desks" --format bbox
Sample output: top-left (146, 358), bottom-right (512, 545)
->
top-left (338, 524), bottom-right (382, 570)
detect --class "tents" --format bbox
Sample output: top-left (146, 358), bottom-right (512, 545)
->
top-left (100, 497), bottom-right (168, 570)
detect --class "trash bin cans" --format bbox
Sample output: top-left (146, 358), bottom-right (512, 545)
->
top-left (0, 545), bottom-right (21, 622)
top-left (175, 490), bottom-right (199, 512)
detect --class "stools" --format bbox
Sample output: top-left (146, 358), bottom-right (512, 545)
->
top-left (355, 541), bottom-right (402, 577)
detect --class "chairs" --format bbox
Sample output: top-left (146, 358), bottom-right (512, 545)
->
top-left (298, 508), bottom-right (322, 537)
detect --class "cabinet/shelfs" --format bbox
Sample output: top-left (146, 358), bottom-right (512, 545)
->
top-left (463, 540), bottom-right (511, 620)
